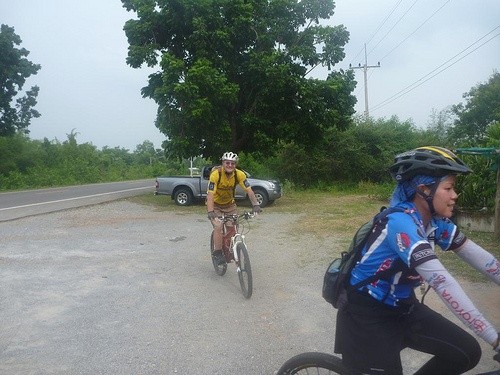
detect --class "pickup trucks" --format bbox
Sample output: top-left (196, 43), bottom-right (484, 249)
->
top-left (154, 165), bottom-right (281, 208)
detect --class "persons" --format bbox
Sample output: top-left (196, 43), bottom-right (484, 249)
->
top-left (334, 146), bottom-right (500, 375)
top-left (204, 152), bottom-right (262, 265)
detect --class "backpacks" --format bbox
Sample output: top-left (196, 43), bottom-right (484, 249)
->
top-left (323, 204), bottom-right (424, 308)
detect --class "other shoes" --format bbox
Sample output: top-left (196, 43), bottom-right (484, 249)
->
top-left (214, 249), bottom-right (225, 265)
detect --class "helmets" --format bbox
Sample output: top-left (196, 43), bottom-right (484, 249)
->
top-left (222, 152), bottom-right (239, 163)
top-left (387, 145), bottom-right (470, 184)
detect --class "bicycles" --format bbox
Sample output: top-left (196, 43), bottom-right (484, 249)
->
top-left (276, 351), bottom-right (500, 375)
top-left (208, 208), bottom-right (263, 298)
